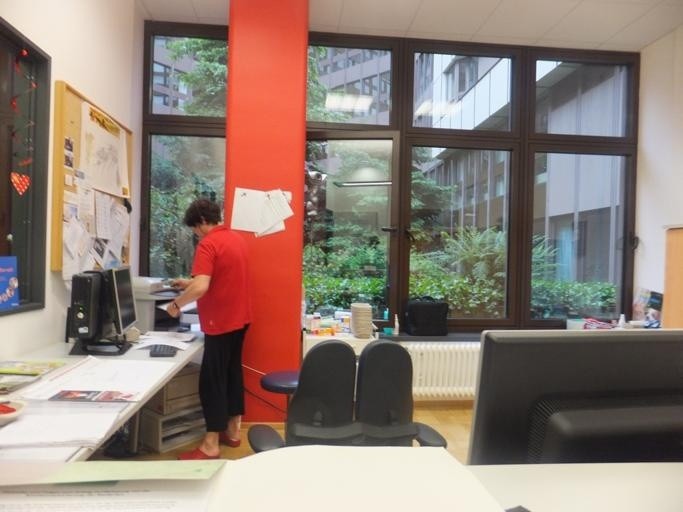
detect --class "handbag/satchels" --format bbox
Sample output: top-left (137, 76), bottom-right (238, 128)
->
top-left (404, 295), bottom-right (451, 338)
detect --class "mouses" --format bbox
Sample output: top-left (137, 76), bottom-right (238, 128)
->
top-left (150, 344), bottom-right (176, 356)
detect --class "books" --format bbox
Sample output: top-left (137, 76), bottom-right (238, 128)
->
top-left (0, 357), bottom-right (176, 463)
top-left (0, 255), bottom-right (20, 307)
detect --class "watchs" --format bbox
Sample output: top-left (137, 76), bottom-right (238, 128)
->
top-left (172, 302), bottom-right (179, 310)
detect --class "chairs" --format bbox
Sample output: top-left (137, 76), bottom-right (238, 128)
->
top-left (248, 339), bottom-right (448, 453)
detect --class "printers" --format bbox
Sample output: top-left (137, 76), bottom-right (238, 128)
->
top-left (130, 275), bottom-right (192, 334)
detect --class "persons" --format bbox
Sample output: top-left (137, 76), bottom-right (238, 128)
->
top-left (166, 200), bottom-right (252, 460)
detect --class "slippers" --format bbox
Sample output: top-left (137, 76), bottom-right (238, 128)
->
top-left (175, 446), bottom-right (221, 462)
top-left (217, 430), bottom-right (242, 449)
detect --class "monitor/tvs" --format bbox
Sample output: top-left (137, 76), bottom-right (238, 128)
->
top-left (108, 265), bottom-right (138, 336)
top-left (467, 327), bottom-right (683, 465)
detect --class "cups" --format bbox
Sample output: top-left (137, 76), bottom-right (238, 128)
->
top-left (383, 310), bottom-right (388, 319)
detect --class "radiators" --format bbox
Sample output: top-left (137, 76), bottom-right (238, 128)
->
top-left (395, 341), bottom-right (482, 400)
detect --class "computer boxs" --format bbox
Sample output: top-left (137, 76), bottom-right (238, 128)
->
top-left (69, 269), bottom-right (112, 339)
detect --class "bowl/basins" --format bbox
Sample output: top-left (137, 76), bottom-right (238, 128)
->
top-left (384, 328), bottom-right (395, 336)
top-left (0, 401), bottom-right (25, 425)
top-left (629, 321), bottom-right (644, 329)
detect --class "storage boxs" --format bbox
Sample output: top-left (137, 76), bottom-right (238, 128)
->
top-left (136, 362), bottom-right (207, 415)
top-left (128, 405), bottom-right (207, 454)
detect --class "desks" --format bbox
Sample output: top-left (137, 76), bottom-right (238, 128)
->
top-left (0, 313), bottom-right (683, 512)
